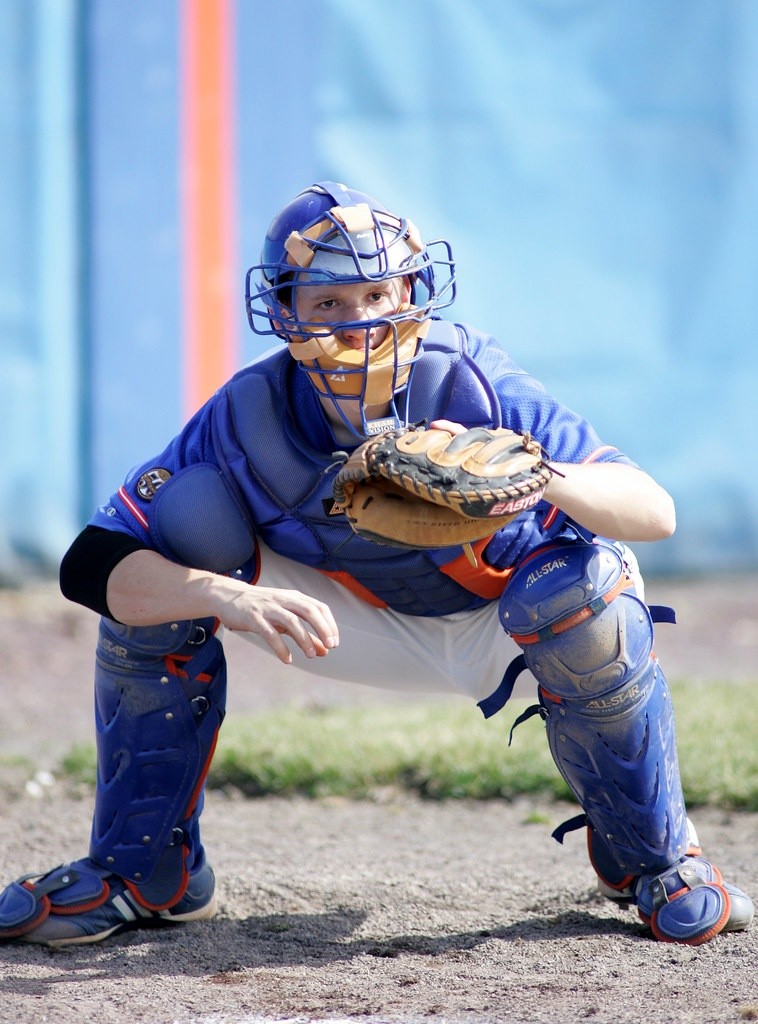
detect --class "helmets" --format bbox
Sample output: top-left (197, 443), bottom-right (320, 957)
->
top-left (244, 182), bottom-right (457, 441)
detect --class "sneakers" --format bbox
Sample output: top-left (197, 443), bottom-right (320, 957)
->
top-left (1, 844), bottom-right (218, 950)
top-left (586, 832), bottom-right (753, 932)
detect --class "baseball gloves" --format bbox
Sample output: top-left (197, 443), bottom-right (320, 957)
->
top-left (332, 419), bottom-right (555, 550)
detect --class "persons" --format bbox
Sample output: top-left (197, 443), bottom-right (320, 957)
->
top-left (0, 181), bottom-right (756, 950)
